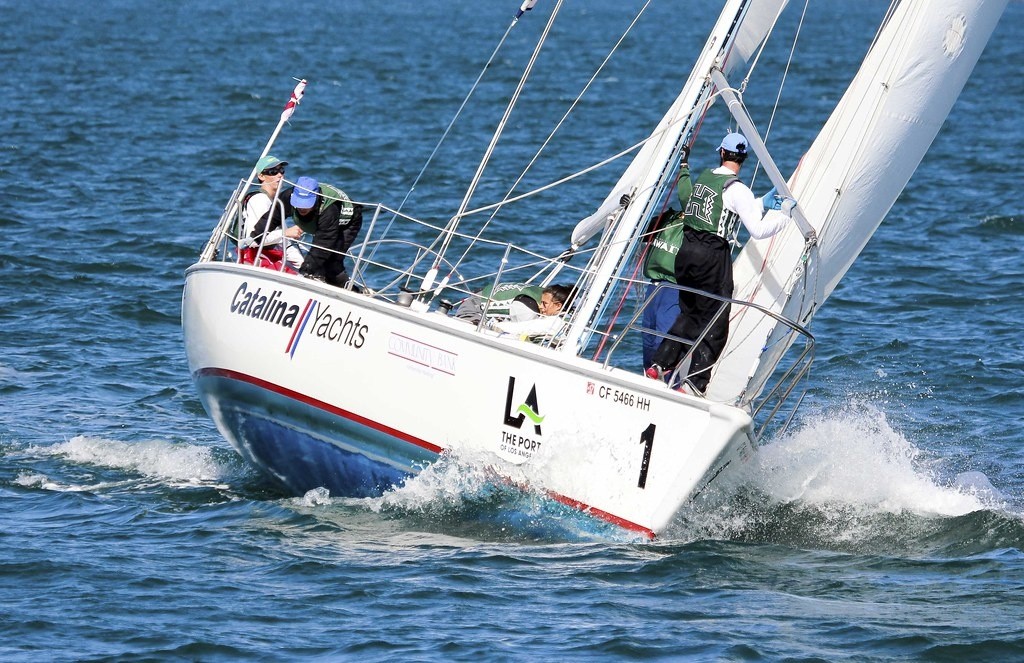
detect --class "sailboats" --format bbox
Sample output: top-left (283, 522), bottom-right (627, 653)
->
top-left (182, 1), bottom-right (1008, 546)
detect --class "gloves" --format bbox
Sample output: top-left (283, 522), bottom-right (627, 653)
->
top-left (680, 145), bottom-right (690, 165)
top-left (619, 194), bottom-right (630, 210)
top-left (238, 237), bottom-right (254, 250)
top-left (761, 186), bottom-right (782, 210)
top-left (780, 198), bottom-right (798, 217)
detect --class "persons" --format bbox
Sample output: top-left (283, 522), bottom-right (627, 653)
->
top-left (456, 282), bottom-right (581, 349)
top-left (645, 132), bottom-right (796, 398)
top-left (229, 155), bottom-right (303, 276)
top-left (238, 177), bottom-right (362, 294)
top-left (619, 143), bottom-right (693, 391)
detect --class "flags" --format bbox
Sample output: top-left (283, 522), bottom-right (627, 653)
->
top-left (280, 79), bottom-right (307, 121)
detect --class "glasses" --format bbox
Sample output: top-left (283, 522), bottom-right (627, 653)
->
top-left (263, 167), bottom-right (284, 176)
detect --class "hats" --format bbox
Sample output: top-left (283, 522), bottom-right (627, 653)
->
top-left (290, 176), bottom-right (319, 208)
top-left (715, 133), bottom-right (748, 153)
top-left (256, 156), bottom-right (288, 175)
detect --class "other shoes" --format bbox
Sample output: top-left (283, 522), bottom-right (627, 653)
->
top-left (677, 382), bottom-right (703, 397)
top-left (648, 365), bottom-right (664, 380)
